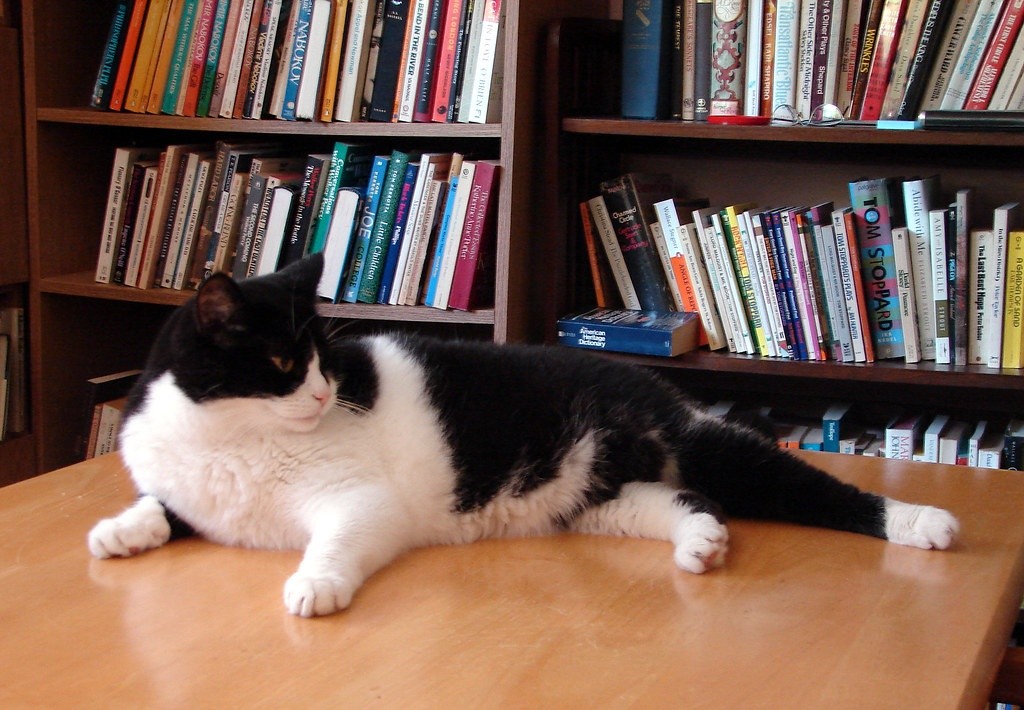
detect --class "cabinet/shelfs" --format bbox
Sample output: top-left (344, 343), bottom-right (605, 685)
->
top-left (0, 0), bottom-right (1024, 475)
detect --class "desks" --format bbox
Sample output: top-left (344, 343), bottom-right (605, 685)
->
top-left (0, 419), bottom-right (1024, 709)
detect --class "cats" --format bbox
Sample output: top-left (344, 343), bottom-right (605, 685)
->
top-left (87, 253), bottom-right (958, 617)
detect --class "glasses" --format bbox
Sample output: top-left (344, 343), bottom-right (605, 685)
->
top-left (769, 103), bottom-right (849, 127)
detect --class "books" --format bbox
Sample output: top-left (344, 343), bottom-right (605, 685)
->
top-left (581, 174), bottom-right (1024, 369)
top-left (0, 307), bottom-right (25, 440)
top-left (95, 141), bottom-right (502, 311)
top-left (558, 307), bottom-right (698, 357)
top-left (90, 0), bottom-right (507, 125)
top-left (73, 368), bottom-right (142, 460)
top-left (709, 398), bottom-right (1024, 472)
top-left (619, 0), bottom-right (1024, 121)
